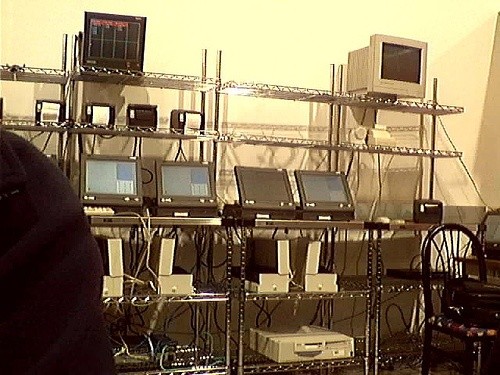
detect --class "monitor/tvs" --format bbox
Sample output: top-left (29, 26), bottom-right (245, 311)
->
top-left (295, 168), bottom-right (356, 212)
top-left (234, 165), bottom-right (297, 211)
top-left (346, 33), bottom-right (429, 101)
top-left (154, 158), bottom-right (218, 208)
top-left (79, 153), bottom-right (143, 207)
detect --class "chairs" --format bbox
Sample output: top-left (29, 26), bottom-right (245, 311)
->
top-left (419, 221), bottom-right (500, 375)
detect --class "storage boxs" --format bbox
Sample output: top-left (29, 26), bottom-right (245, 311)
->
top-left (248, 323), bottom-right (356, 362)
top-left (230, 237), bottom-right (291, 293)
top-left (294, 236), bottom-right (340, 293)
top-left (155, 237), bottom-right (195, 295)
top-left (94, 233), bottom-right (125, 298)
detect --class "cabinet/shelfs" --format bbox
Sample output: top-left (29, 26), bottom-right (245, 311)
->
top-left (0, 33), bottom-right (69, 174)
top-left (205, 48), bottom-right (373, 375)
top-left (66, 29), bottom-right (232, 375)
top-left (327, 63), bottom-right (464, 375)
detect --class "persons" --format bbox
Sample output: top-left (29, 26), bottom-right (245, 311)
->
top-left (0, 129), bottom-right (116, 375)
